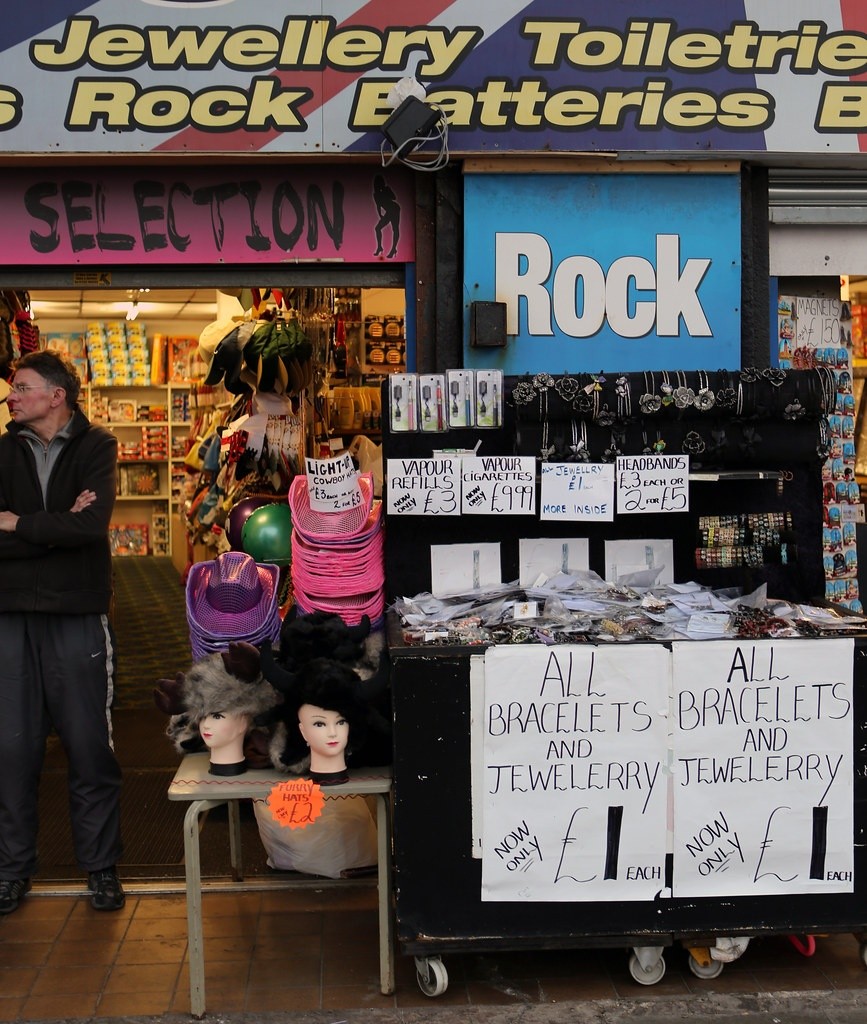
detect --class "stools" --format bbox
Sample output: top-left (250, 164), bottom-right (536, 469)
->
top-left (168, 750), bottom-right (397, 1022)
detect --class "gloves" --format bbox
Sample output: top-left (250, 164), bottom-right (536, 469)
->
top-left (218, 412), bottom-right (303, 477)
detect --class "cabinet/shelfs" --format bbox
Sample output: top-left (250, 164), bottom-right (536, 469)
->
top-left (390, 600), bottom-right (867, 996)
top-left (88, 385), bottom-right (173, 558)
top-left (170, 383), bottom-right (190, 504)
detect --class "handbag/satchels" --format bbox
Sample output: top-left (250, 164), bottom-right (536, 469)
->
top-left (183, 398), bottom-right (248, 474)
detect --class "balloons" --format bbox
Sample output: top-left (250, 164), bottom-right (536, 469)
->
top-left (240, 503), bottom-right (293, 568)
top-left (224, 499), bottom-right (272, 552)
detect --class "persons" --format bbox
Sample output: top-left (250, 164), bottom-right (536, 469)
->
top-left (157, 640), bottom-right (278, 777)
top-left (259, 604), bottom-right (384, 785)
top-left (0, 351), bottom-right (126, 912)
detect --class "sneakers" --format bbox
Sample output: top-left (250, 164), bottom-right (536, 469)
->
top-left (88, 866), bottom-right (127, 911)
top-left (1, 879), bottom-right (33, 913)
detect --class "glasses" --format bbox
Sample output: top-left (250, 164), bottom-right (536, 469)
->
top-left (9, 383), bottom-right (58, 393)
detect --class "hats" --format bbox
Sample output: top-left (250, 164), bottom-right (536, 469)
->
top-left (294, 657), bottom-right (369, 729)
top-left (195, 317), bottom-right (315, 396)
top-left (151, 640), bottom-right (306, 724)
top-left (184, 551), bottom-right (282, 664)
top-left (288, 469), bottom-right (386, 630)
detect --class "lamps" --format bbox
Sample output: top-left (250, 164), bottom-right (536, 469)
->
top-left (124, 290), bottom-right (149, 323)
top-left (382, 98), bottom-right (444, 159)
